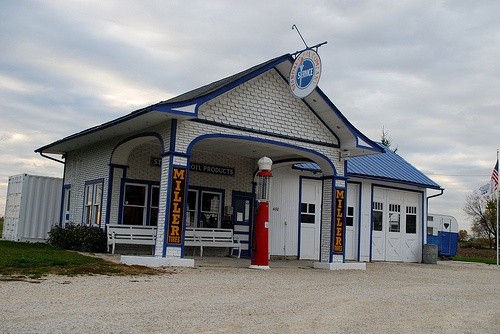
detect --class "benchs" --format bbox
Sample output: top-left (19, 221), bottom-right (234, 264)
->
top-left (105, 224), bottom-right (242, 260)
